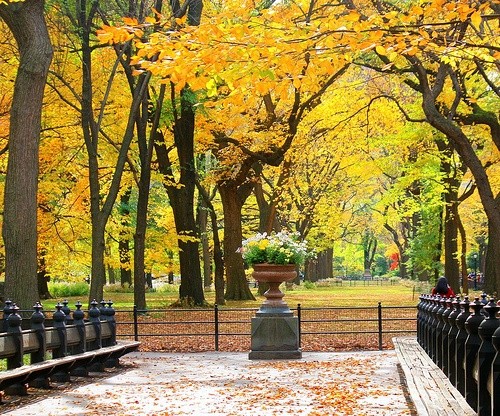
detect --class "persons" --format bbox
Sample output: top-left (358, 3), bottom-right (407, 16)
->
top-left (432, 277), bottom-right (456, 298)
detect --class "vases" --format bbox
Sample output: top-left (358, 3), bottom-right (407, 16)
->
top-left (251, 263), bottom-right (299, 305)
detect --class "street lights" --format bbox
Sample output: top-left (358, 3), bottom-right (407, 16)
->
top-left (473, 252), bottom-right (479, 291)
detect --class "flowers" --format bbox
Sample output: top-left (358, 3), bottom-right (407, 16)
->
top-left (235, 229), bottom-right (321, 265)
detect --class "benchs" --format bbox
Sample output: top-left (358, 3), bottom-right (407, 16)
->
top-left (0, 300), bottom-right (141, 407)
